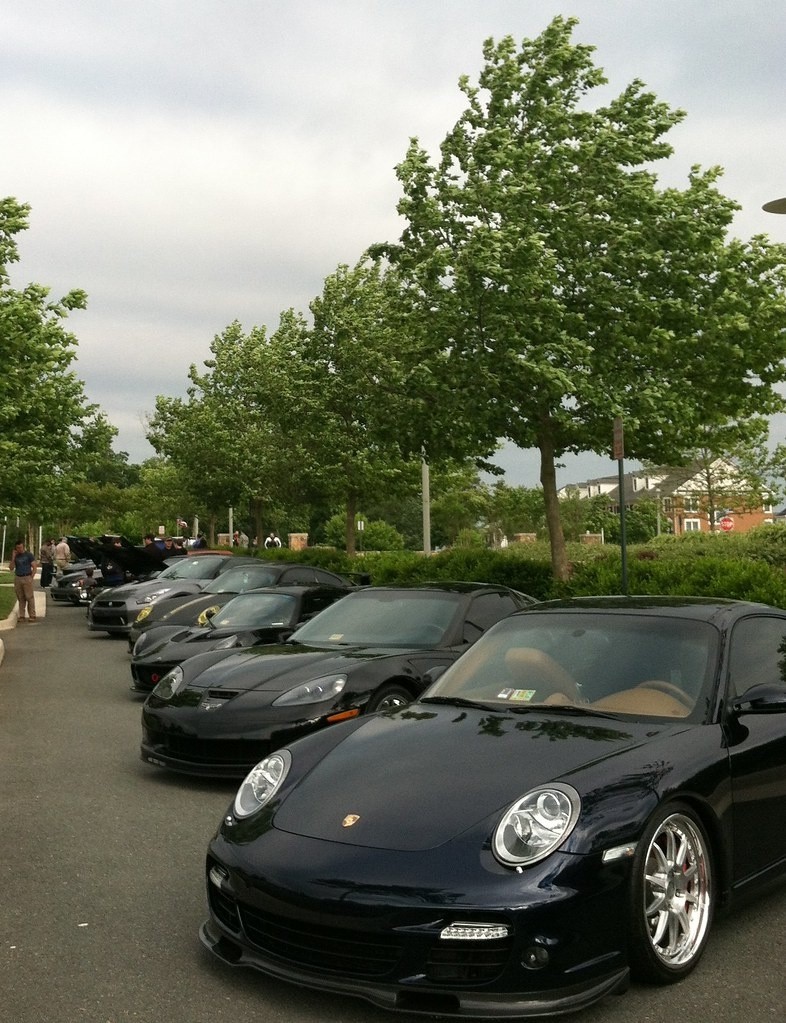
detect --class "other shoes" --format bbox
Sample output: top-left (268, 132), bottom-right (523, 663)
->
top-left (18, 617), bottom-right (25, 622)
top-left (29, 616), bottom-right (35, 621)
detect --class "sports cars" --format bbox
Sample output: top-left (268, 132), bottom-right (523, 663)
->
top-left (194, 593), bottom-right (786, 1023)
top-left (136, 579), bottom-right (543, 781)
top-left (49, 530), bottom-right (374, 696)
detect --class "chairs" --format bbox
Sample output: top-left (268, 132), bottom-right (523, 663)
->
top-left (504, 646), bottom-right (579, 703)
top-left (664, 636), bottom-right (708, 696)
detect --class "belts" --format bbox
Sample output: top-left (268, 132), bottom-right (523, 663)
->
top-left (17, 573), bottom-right (31, 577)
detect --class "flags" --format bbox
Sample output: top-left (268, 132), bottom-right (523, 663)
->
top-left (176, 518), bottom-right (187, 528)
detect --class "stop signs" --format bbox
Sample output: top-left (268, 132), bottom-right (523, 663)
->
top-left (719, 516), bottom-right (734, 531)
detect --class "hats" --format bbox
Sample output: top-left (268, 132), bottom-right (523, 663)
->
top-left (166, 537), bottom-right (171, 541)
top-left (143, 534), bottom-right (154, 539)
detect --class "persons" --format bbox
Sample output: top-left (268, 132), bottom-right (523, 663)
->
top-left (8, 541), bottom-right (38, 622)
top-left (83, 536), bottom-right (207, 590)
top-left (234, 530), bottom-right (282, 548)
top-left (41, 537), bottom-right (70, 589)
